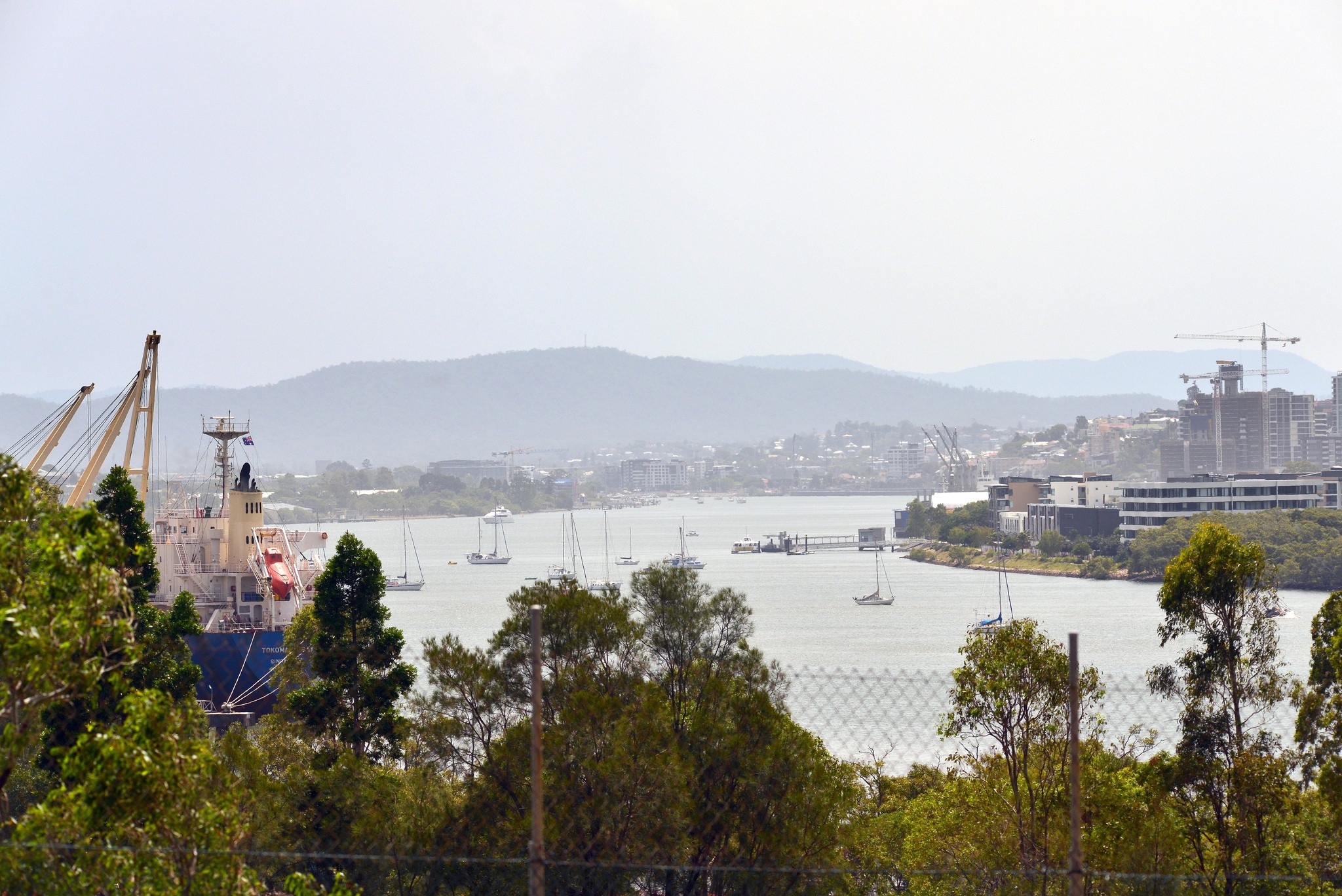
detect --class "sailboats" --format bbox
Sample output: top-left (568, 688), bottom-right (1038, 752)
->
top-left (974, 526), bottom-right (1017, 635)
top-left (852, 535), bottom-right (895, 605)
top-left (464, 496), bottom-right (513, 565)
top-left (583, 510), bottom-right (623, 590)
top-left (382, 506), bottom-right (425, 591)
top-left (529, 512), bottom-right (589, 596)
top-left (662, 515), bottom-right (708, 570)
top-left (614, 527), bottom-right (640, 565)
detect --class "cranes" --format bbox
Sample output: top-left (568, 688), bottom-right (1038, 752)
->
top-left (491, 447), bottom-right (570, 483)
top-left (1173, 321), bottom-right (1301, 474)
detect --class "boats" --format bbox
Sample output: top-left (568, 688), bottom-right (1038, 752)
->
top-left (482, 499), bottom-right (515, 524)
top-left (666, 496), bottom-right (674, 501)
top-left (713, 495), bottom-right (723, 500)
top-left (731, 526), bottom-right (761, 553)
top-left (685, 530), bottom-right (700, 537)
top-left (727, 496), bottom-right (746, 503)
top-left (689, 496), bottom-right (704, 504)
top-left (1249, 579), bottom-right (1301, 620)
top-left (151, 408), bottom-right (329, 733)
